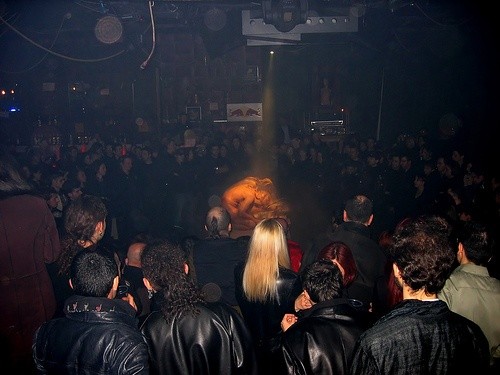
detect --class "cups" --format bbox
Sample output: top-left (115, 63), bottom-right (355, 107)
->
top-left (116, 285), bottom-right (129, 303)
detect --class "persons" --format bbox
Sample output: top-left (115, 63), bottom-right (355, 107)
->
top-left (347, 212), bottom-right (492, 375)
top-left (136, 237), bottom-right (259, 374)
top-left (435, 220), bottom-right (500, 375)
top-left (29, 246), bottom-right (150, 374)
top-left (0, 103), bottom-right (500, 329)
top-left (233, 217), bottom-right (302, 374)
top-left (267, 257), bottom-right (375, 375)
top-left (0, 150), bottom-right (63, 375)
